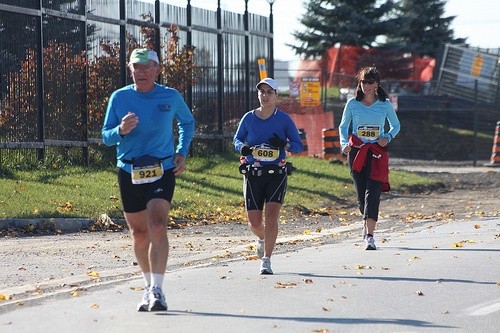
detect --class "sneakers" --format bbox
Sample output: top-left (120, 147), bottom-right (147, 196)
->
top-left (149, 287), bottom-right (167, 312)
top-left (137, 289), bottom-right (151, 312)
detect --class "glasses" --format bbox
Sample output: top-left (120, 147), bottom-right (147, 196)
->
top-left (132, 66), bottom-right (153, 73)
top-left (362, 80), bottom-right (375, 84)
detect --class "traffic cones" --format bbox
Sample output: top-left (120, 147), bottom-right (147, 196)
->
top-left (482, 120), bottom-right (500, 167)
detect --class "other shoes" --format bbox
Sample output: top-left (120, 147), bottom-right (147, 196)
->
top-left (260, 257), bottom-right (273, 274)
top-left (256, 239), bottom-right (266, 259)
top-left (365, 236), bottom-right (376, 250)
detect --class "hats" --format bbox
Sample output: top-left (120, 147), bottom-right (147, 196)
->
top-left (127, 48), bottom-right (160, 67)
top-left (256, 78), bottom-right (277, 90)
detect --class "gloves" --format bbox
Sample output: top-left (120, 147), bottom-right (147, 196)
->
top-left (242, 146), bottom-right (253, 156)
top-left (268, 132), bottom-right (287, 149)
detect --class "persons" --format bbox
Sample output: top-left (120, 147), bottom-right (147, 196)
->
top-left (339, 67), bottom-right (400, 250)
top-left (233, 78), bottom-right (304, 275)
top-left (102, 47), bottom-right (194, 311)
